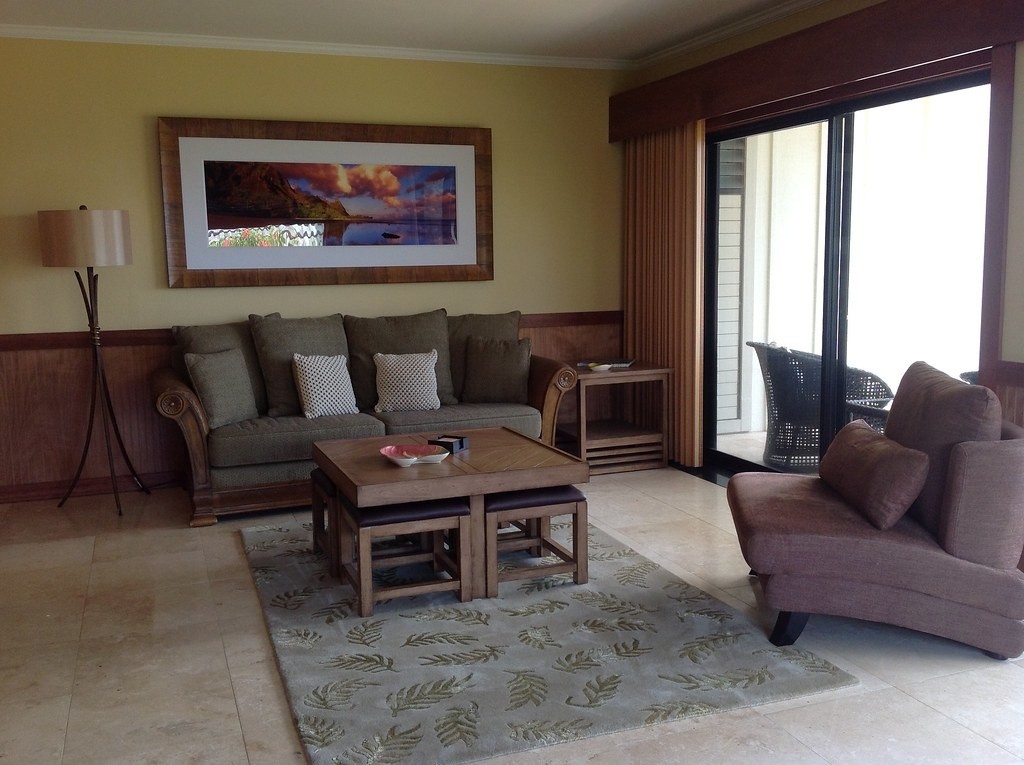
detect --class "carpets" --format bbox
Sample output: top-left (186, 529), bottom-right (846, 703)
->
top-left (240, 510), bottom-right (860, 764)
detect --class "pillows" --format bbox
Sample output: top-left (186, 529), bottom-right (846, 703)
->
top-left (169, 311), bottom-right (282, 404)
top-left (459, 335), bottom-right (533, 404)
top-left (817, 419), bottom-right (930, 531)
top-left (345, 308), bottom-right (459, 410)
top-left (883, 361), bottom-right (1002, 537)
top-left (183, 347), bottom-right (260, 428)
top-left (447, 310), bottom-right (522, 399)
top-left (288, 353), bottom-right (360, 418)
top-left (371, 349), bottom-right (442, 410)
top-left (247, 314), bottom-right (351, 418)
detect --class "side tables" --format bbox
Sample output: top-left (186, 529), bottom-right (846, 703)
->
top-left (558, 359), bottom-right (675, 478)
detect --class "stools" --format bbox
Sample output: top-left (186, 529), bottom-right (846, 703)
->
top-left (312, 467), bottom-right (587, 617)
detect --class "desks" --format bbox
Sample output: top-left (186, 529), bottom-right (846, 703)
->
top-left (847, 396), bottom-right (894, 426)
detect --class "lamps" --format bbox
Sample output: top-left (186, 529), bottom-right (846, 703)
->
top-left (35, 205), bottom-right (153, 518)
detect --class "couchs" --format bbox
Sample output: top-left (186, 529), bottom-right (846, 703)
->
top-left (726, 422), bottom-right (1022, 661)
top-left (150, 352), bottom-right (578, 532)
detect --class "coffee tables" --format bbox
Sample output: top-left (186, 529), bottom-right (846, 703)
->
top-left (311, 426), bottom-right (590, 599)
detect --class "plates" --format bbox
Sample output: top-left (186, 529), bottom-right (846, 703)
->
top-left (588, 364), bottom-right (611, 371)
top-left (379, 444), bottom-right (450, 467)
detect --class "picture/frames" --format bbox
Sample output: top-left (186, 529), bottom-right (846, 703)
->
top-left (156, 113), bottom-right (494, 289)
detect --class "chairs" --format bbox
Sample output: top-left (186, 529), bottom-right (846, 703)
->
top-left (746, 340), bottom-right (894, 477)
